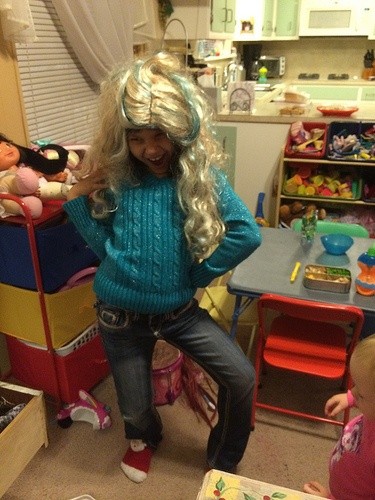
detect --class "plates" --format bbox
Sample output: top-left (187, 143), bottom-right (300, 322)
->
top-left (317, 105), bottom-right (358, 116)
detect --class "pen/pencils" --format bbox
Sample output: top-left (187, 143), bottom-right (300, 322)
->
top-left (290, 262), bottom-right (301, 282)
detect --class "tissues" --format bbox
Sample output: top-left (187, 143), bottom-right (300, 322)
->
top-left (195, 73), bottom-right (226, 115)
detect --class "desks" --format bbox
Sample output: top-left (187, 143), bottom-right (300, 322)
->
top-left (225, 226), bottom-right (374, 341)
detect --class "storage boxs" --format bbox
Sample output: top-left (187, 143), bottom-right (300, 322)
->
top-left (0, 380), bottom-right (49, 500)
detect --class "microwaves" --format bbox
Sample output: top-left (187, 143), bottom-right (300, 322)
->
top-left (251, 55), bottom-right (287, 78)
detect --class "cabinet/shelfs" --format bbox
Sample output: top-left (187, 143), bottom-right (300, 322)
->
top-left (163, 0), bottom-right (375, 88)
top-left (272, 121), bottom-right (375, 238)
top-left (0, 143), bottom-right (115, 430)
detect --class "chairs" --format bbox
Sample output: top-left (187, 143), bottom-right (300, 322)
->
top-left (292, 219), bottom-right (370, 238)
top-left (249, 293), bottom-right (366, 439)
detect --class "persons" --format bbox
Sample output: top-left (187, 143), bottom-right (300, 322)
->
top-left (65, 51), bottom-right (262, 482)
top-left (304, 332), bottom-right (375, 500)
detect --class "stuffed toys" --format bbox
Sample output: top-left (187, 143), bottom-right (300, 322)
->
top-left (0, 132), bottom-right (82, 218)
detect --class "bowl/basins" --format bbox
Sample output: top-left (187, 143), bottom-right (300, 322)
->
top-left (303, 263), bottom-right (351, 293)
top-left (320, 233), bottom-right (353, 255)
